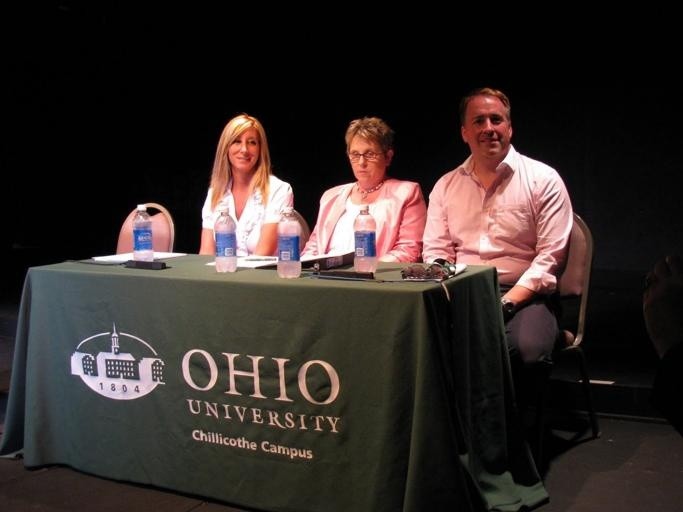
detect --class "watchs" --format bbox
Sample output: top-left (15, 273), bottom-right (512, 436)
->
top-left (501, 296), bottom-right (516, 316)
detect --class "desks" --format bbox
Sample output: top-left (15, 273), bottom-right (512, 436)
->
top-left (28, 252), bottom-right (497, 507)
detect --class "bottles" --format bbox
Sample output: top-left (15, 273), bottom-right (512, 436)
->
top-left (353, 203), bottom-right (378, 276)
top-left (132, 205), bottom-right (154, 262)
top-left (213, 206), bottom-right (239, 272)
top-left (276, 206), bottom-right (302, 279)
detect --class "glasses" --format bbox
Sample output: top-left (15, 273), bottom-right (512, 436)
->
top-left (347, 152), bottom-right (382, 160)
top-left (400, 258), bottom-right (456, 279)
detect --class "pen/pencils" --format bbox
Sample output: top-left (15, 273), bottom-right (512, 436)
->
top-left (244, 258), bottom-right (276, 261)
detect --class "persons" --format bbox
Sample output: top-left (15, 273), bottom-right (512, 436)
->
top-left (421, 87), bottom-right (576, 420)
top-left (299, 114), bottom-right (427, 266)
top-left (640, 254), bottom-right (683, 438)
top-left (197, 110), bottom-right (296, 258)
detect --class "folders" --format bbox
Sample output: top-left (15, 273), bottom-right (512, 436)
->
top-left (255, 251), bottom-right (356, 271)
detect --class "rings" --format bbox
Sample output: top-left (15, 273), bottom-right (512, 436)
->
top-left (640, 272), bottom-right (655, 293)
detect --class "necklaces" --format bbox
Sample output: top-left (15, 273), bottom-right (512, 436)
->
top-left (356, 183), bottom-right (384, 199)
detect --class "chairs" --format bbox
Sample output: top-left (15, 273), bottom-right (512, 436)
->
top-left (117, 203), bottom-right (308, 255)
top-left (537, 211), bottom-right (600, 469)
